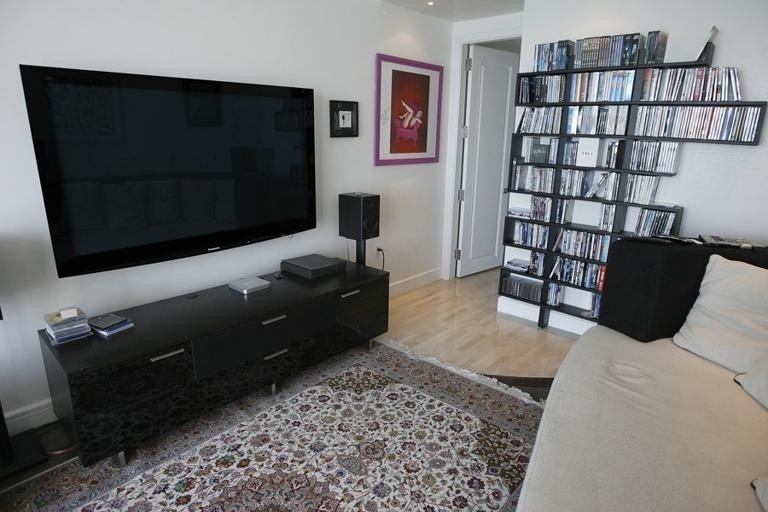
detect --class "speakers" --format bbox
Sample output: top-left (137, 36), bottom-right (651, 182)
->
top-left (339, 192), bottom-right (380, 240)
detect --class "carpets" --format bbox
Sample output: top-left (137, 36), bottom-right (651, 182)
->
top-left (0, 334), bottom-right (548, 511)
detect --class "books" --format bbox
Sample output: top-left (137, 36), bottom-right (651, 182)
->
top-left (41, 305), bottom-right (135, 347)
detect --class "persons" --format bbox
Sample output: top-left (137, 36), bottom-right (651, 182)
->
top-left (398, 99), bottom-right (423, 128)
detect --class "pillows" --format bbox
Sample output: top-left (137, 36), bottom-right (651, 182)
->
top-left (672, 254), bottom-right (768, 411)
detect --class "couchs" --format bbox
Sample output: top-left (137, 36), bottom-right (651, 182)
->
top-left (513, 236), bottom-right (768, 512)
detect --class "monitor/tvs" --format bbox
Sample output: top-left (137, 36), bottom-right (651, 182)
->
top-left (19, 64), bottom-right (316, 279)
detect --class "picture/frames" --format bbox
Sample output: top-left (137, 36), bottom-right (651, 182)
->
top-left (328, 53), bottom-right (445, 168)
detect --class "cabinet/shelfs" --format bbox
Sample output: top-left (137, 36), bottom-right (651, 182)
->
top-left (37, 256), bottom-right (390, 468)
top-left (498, 42), bottom-right (767, 328)
